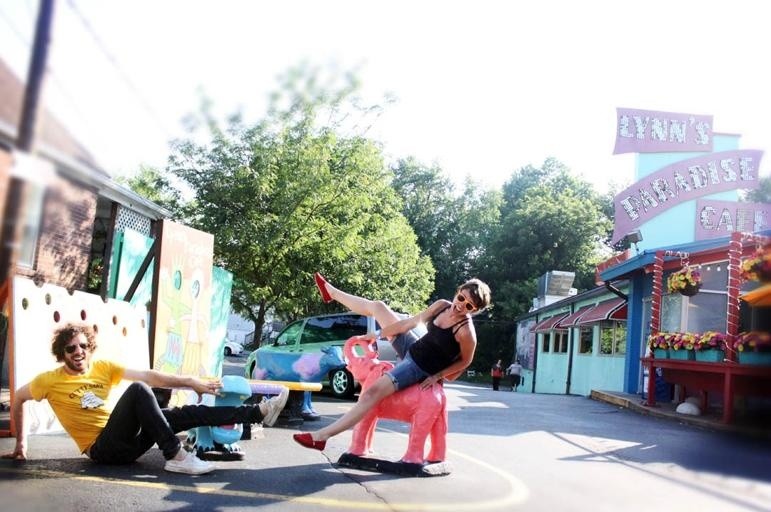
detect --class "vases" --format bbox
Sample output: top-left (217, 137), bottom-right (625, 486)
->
top-left (653, 348), bottom-right (670, 358)
top-left (738, 351), bottom-right (771, 364)
top-left (695, 349), bottom-right (724, 362)
top-left (670, 349), bottom-right (695, 360)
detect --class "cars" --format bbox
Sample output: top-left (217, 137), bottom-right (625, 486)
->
top-left (224, 337), bottom-right (244, 357)
top-left (243, 312), bottom-right (429, 400)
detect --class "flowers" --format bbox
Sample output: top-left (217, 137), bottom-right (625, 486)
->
top-left (665, 330), bottom-right (696, 351)
top-left (734, 331), bottom-right (771, 352)
top-left (650, 331), bottom-right (671, 349)
top-left (667, 266), bottom-right (703, 295)
top-left (742, 249), bottom-right (771, 283)
top-left (693, 331), bottom-right (727, 351)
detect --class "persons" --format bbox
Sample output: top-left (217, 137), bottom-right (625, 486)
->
top-left (291, 272), bottom-right (491, 451)
top-left (491, 359), bottom-right (504, 391)
top-left (3, 323), bottom-right (289, 475)
top-left (506, 360), bottom-right (523, 392)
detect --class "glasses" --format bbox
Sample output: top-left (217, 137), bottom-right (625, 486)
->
top-left (457, 294), bottom-right (479, 314)
top-left (64, 343), bottom-right (92, 352)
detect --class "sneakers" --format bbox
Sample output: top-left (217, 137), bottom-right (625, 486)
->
top-left (164, 451), bottom-right (216, 475)
top-left (262, 388), bottom-right (289, 427)
top-left (294, 432), bottom-right (327, 452)
top-left (314, 272), bottom-right (331, 304)
